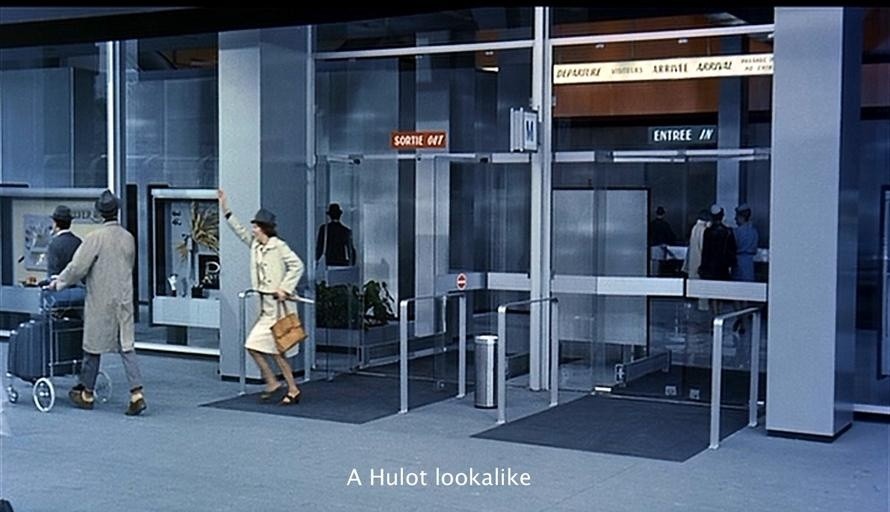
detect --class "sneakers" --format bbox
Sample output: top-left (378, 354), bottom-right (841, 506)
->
top-left (69, 391), bottom-right (94, 408)
top-left (126, 397), bottom-right (147, 415)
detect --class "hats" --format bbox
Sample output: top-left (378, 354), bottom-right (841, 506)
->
top-left (96, 190), bottom-right (122, 216)
top-left (326, 204), bottom-right (342, 213)
top-left (50, 206), bottom-right (75, 220)
top-left (251, 209), bottom-right (275, 227)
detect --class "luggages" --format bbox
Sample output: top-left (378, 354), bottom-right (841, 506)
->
top-left (8, 286), bottom-right (86, 379)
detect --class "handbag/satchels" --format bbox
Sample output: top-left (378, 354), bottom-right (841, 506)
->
top-left (315, 255), bottom-right (328, 287)
top-left (270, 313), bottom-right (307, 353)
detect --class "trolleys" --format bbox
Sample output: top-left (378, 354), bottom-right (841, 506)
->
top-left (5, 273), bottom-right (114, 411)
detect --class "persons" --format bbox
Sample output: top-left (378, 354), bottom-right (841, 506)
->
top-left (44, 204), bottom-right (82, 280)
top-left (316, 204), bottom-right (356, 267)
top-left (49, 190), bottom-right (147, 415)
top-left (648, 205), bottom-right (674, 277)
top-left (683, 202), bottom-right (759, 336)
top-left (217, 187), bottom-right (304, 406)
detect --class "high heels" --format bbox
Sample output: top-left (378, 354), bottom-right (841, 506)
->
top-left (281, 389), bottom-right (302, 405)
top-left (261, 384), bottom-right (281, 399)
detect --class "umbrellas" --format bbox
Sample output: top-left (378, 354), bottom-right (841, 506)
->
top-left (244, 288), bottom-right (316, 305)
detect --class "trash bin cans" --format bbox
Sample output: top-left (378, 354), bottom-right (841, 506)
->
top-left (474, 335), bottom-right (498, 408)
